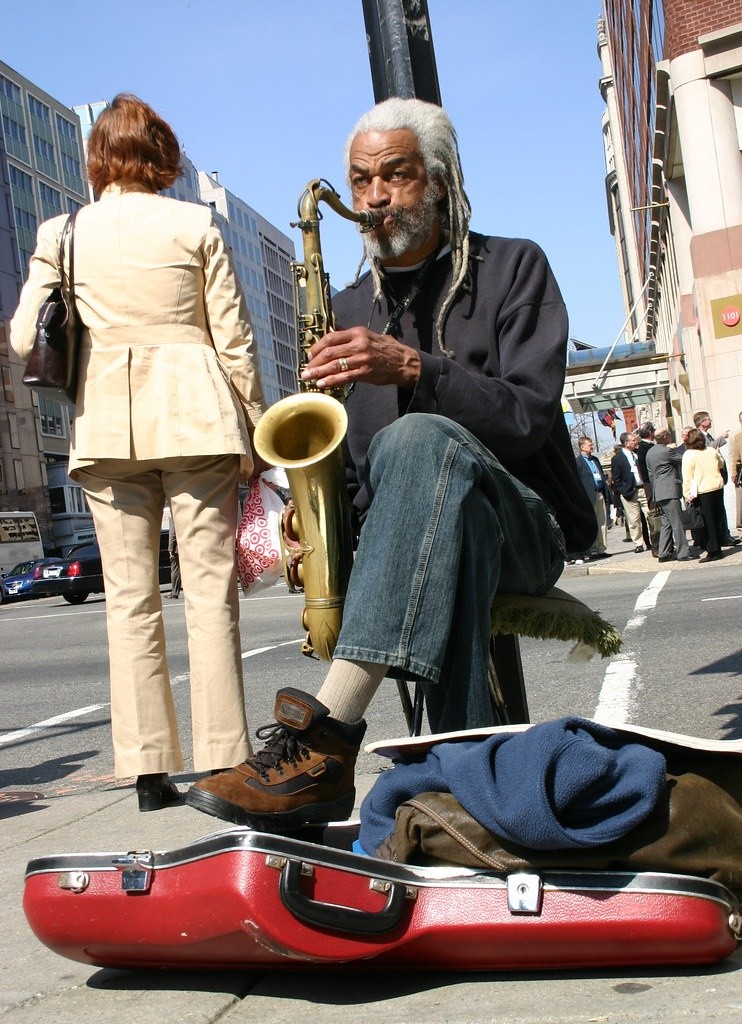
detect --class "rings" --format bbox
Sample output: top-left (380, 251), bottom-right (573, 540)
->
top-left (338, 357), bottom-right (348, 372)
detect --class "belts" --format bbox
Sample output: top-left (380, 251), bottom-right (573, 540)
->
top-left (595, 489), bottom-right (602, 492)
top-left (634, 485), bottom-right (644, 489)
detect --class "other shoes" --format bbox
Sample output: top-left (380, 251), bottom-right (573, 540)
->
top-left (722, 537), bottom-right (741, 546)
top-left (651, 548), bottom-right (659, 558)
top-left (659, 554), bottom-right (678, 562)
top-left (575, 559), bottom-right (584, 565)
top-left (634, 545), bottom-right (644, 553)
top-left (693, 540), bottom-right (700, 546)
top-left (698, 552), bottom-right (724, 562)
top-left (678, 553), bottom-right (700, 561)
top-left (623, 538), bottom-right (632, 542)
top-left (589, 551), bottom-right (612, 559)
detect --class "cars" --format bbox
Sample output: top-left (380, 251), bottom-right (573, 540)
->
top-left (30, 529), bottom-right (172, 605)
top-left (0, 557), bottom-right (64, 602)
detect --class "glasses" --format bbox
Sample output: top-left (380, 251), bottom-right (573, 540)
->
top-left (701, 417), bottom-right (711, 422)
top-left (625, 439), bottom-right (636, 442)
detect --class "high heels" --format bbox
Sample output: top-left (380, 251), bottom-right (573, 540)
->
top-left (135, 772), bottom-right (180, 813)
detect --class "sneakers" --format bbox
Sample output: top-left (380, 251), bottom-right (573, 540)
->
top-left (185, 686), bottom-right (367, 830)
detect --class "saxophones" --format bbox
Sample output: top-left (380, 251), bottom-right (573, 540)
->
top-left (253, 179), bottom-right (386, 660)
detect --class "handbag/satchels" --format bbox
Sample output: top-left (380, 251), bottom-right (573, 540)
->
top-left (734, 463), bottom-right (742, 487)
top-left (647, 502), bottom-right (661, 534)
top-left (680, 502), bottom-right (704, 529)
top-left (235, 465), bottom-right (292, 595)
top-left (20, 210), bottom-right (81, 407)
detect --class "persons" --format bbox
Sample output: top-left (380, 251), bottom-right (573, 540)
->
top-left (8, 90), bottom-right (268, 811)
top-left (577, 409), bottom-right (742, 564)
top-left (184, 94), bottom-right (599, 829)
